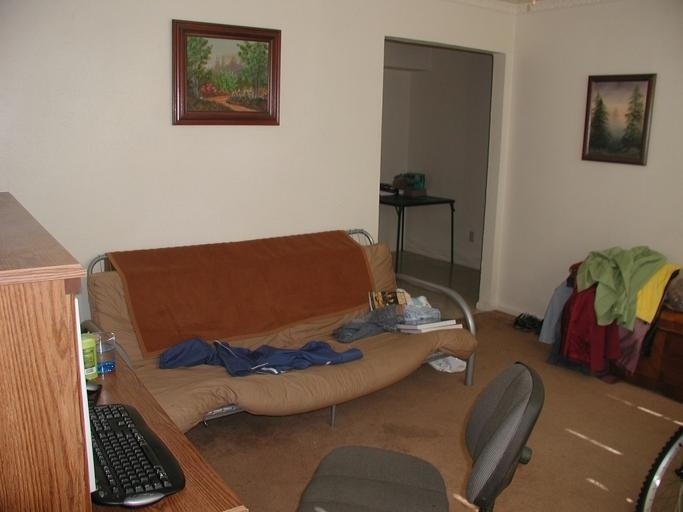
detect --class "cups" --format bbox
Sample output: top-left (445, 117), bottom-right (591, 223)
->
top-left (89, 330), bottom-right (116, 374)
top-left (78, 333), bottom-right (98, 379)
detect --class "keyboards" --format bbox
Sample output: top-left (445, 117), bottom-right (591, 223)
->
top-left (89, 404), bottom-right (186, 509)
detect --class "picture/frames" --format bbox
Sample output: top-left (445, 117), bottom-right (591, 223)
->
top-left (581, 74), bottom-right (656, 165)
top-left (172, 19), bottom-right (281, 125)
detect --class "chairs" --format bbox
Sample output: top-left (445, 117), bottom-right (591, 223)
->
top-left (295, 362), bottom-right (545, 512)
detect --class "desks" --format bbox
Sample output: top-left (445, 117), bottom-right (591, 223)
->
top-left (380, 195), bottom-right (455, 272)
top-left (87, 352), bottom-right (248, 512)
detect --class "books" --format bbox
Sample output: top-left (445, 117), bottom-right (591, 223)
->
top-left (367, 290), bottom-right (464, 334)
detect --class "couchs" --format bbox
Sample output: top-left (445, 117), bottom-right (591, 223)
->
top-left (81, 229), bottom-right (478, 434)
